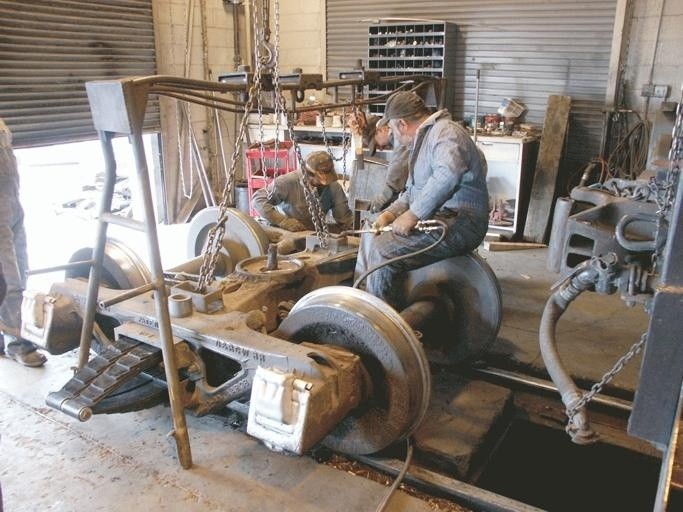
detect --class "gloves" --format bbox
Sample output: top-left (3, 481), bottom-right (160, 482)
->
top-left (264, 207), bottom-right (306, 232)
top-left (392, 208), bottom-right (418, 239)
top-left (372, 210), bottom-right (394, 235)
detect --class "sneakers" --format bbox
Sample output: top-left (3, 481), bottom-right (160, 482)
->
top-left (3, 339), bottom-right (47, 367)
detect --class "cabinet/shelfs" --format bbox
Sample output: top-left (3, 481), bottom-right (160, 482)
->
top-left (247, 125), bottom-right (362, 224)
top-left (367, 22), bottom-right (457, 118)
top-left (245, 141), bottom-right (297, 216)
top-left (471, 136), bottom-right (538, 241)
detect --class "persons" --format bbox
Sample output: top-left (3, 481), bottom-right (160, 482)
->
top-left (0, 117), bottom-right (48, 366)
top-left (361, 113), bottom-right (494, 215)
top-left (251, 150), bottom-right (354, 236)
top-left (350, 89), bottom-right (489, 313)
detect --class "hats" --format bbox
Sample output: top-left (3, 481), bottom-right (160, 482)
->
top-left (363, 115), bottom-right (381, 157)
top-left (304, 151), bottom-right (338, 186)
top-left (375, 91), bottom-right (426, 130)
